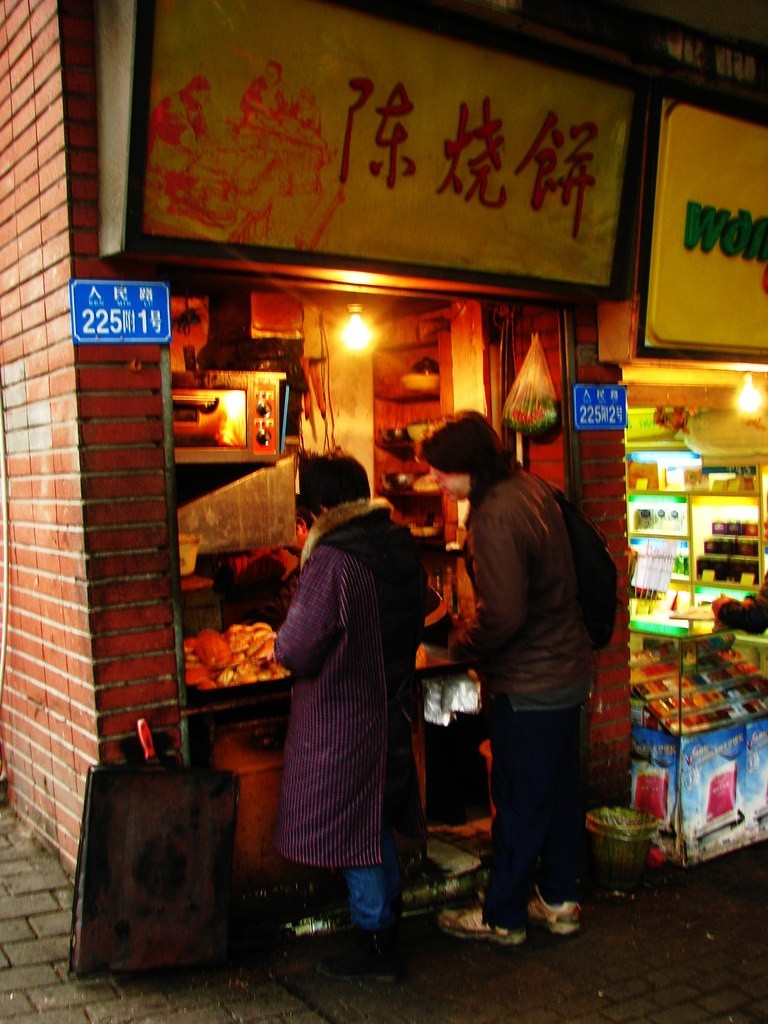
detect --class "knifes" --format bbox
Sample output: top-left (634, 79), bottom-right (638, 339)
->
top-left (137, 718), bottom-right (160, 765)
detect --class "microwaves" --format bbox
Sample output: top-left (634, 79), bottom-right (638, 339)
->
top-left (172, 372), bottom-right (291, 465)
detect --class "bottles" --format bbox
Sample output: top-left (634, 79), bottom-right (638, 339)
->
top-left (634, 509), bottom-right (684, 532)
top-left (634, 588), bottom-right (667, 615)
top-left (672, 541), bottom-right (689, 575)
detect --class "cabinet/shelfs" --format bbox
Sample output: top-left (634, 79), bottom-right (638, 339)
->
top-left (624, 441), bottom-right (768, 683)
top-left (629, 618), bottom-right (768, 869)
top-left (371, 331), bottom-right (459, 624)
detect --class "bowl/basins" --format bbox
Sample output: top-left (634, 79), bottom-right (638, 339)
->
top-left (381, 428), bottom-right (412, 443)
top-left (401, 374), bottom-right (439, 390)
top-left (407, 424), bottom-right (428, 441)
top-left (381, 473), bottom-right (425, 490)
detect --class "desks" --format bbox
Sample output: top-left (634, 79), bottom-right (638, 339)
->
top-left (185, 642), bottom-right (496, 952)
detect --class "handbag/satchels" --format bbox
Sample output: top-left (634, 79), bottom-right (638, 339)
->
top-left (501, 329), bottom-right (560, 438)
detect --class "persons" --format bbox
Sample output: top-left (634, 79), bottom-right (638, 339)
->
top-left (422, 412), bottom-right (597, 946)
top-left (273, 456), bottom-right (427, 974)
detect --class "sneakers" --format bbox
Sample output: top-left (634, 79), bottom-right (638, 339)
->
top-left (525, 881), bottom-right (582, 936)
top-left (437, 889), bottom-right (527, 948)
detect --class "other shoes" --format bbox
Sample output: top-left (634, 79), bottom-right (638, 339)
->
top-left (321, 934), bottom-right (401, 985)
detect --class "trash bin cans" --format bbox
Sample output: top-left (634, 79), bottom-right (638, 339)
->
top-left (584, 804), bottom-right (658, 889)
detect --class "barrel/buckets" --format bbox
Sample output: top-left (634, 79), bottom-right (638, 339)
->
top-left (214, 732), bottom-right (286, 883)
